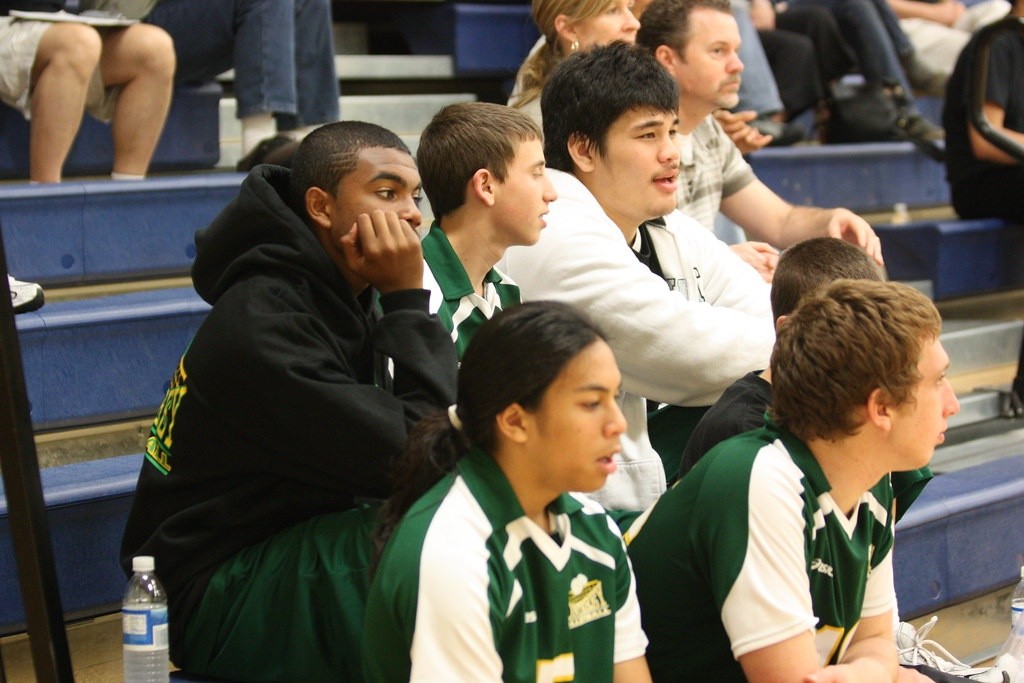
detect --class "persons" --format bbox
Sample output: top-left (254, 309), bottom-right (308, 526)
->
top-left (0, 1), bottom-right (1023, 683)
top-left (623, 282), bottom-right (959, 683)
top-left (364, 303), bottom-right (653, 683)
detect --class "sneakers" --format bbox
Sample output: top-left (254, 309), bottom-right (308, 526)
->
top-left (898, 614), bottom-right (1009, 683)
top-left (7, 275), bottom-right (45, 313)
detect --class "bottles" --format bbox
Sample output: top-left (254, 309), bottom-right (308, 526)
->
top-left (123, 555), bottom-right (169, 683)
top-left (890, 202), bottom-right (912, 226)
top-left (1010, 567), bottom-right (1023, 638)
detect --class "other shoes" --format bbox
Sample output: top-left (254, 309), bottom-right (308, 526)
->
top-left (236, 132), bottom-right (297, 173)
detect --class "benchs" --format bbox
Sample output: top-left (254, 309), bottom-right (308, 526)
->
top-left (0, 0), bottom-right (1024, 639)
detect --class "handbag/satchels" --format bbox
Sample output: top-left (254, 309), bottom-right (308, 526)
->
top-left (823, 72), bottom-right (909, 140)
